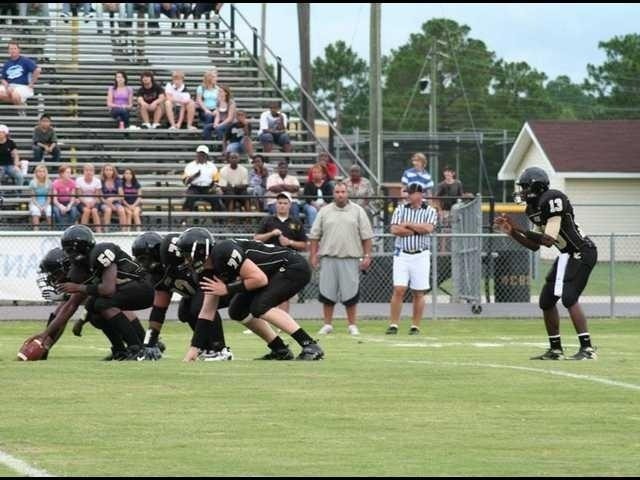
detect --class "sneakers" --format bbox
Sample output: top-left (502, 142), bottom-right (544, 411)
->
top-left (349, 324), bottom-right (359, 336)
top-left (256, 345), bottom-right (293, 359)
top-left (386, 326), bottom-right (396, 334)
top-left (409, 328), bottom-right (418, 335)
top-left (198, 346), bottom-right (233, 360)
top-left (318, 324), bottom-right (332, 334)
top-left (296, 339), bottom-right (324, 360)
top-left (531, 348), bottom-right (565, 360)
top-left (111, 342), bottom-right (165, 361)
top-left (566, 346), bottom-right (596, 360)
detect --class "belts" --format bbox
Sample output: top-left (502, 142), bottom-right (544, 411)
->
top-left (403, 248), bottom-right (428, 254)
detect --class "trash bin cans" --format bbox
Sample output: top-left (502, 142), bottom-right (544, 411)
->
top-left (493, 249), bottom-right (531, 303)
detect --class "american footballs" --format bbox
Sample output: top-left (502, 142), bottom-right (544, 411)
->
top-left (16, 337), bottom-right (51, 361)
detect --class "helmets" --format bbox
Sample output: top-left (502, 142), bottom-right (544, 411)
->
top-left (511, 167), bottom-right (549, 201)
top-left (39, 225), bottom-right (95, 281)
top-left (132, 227), bottom-right (216, 266)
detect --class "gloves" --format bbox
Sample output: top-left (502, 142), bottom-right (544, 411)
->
top-left (42, 286), bottom-right (69, 301)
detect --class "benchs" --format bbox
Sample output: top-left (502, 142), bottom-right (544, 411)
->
top-left (0, 2), bottom-right (347, 237)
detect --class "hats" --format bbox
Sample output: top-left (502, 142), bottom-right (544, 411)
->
top-left (276, 191), bottom-right (292, 202)
top-left (403, 183), bottom-right (422, 192)
top-left (196, 146), bottom-right (208, 154)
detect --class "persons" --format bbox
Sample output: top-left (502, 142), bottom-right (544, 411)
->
top-left (181, 146), bottom-right (375, 229)
top-left (107, 71), bottom-right (254, 159)
top-left (311, 183), bottom-right (374, 338)
top-left (60, 3), bottom-right (223, 37)
top-left (400, 153), bottom-right (465, 251)
top-left (1, 1), bottom-right (51, 32)
top-left (492, 169), bottom-right (599, 362)
top-left (258, 103), bottom-right (293, 165)
top-left (384, 184), bottom-right (439, 335)
top-left (241, 191), bottom-right (311, 337)
top-left (0, 114), bottom-right (144, 232)
top-left (131, 228), bottom-right (324, 359)
top-left (25, 224), bottom-right (165, 359)
top-left (1, 41), bottom-right (41, 117)
